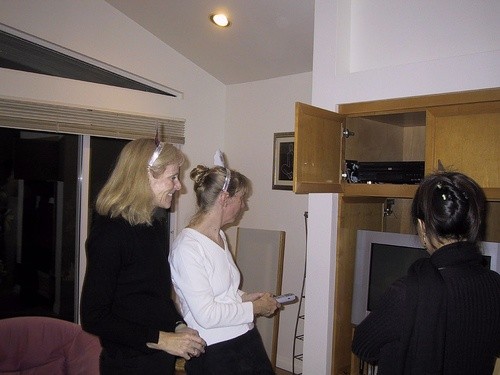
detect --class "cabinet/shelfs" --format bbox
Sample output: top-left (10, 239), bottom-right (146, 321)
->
top-left (292, 85), bottom-right (500, 375)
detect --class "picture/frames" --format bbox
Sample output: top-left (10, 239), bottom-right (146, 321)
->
top-left (272, 131), bottom-right (295, 191)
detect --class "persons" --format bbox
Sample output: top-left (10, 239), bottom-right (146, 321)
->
top-left (352, 168), bottom-right (500, 375)
top-left (167, 163), bottom-right (283, 374)
top-left (81, 133), bottom-right (208, 375)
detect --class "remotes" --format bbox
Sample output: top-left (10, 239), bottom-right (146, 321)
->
top-left (274, 293), bottom-right (295, 303)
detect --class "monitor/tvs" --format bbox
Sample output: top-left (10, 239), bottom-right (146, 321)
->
top-left (351, 230), bottom-right (500, 326)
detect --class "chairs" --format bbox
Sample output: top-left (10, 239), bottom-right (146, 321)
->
top-left (174, 226), bottom-right (287, 370)
top-left (0, 315), bottom-right (103, 375)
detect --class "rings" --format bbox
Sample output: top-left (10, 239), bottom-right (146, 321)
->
top-left (192, 348), bottom-right (195, 356)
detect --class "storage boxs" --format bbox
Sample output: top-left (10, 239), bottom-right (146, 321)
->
top-left (345, 158), bottom-right (425, 186)
